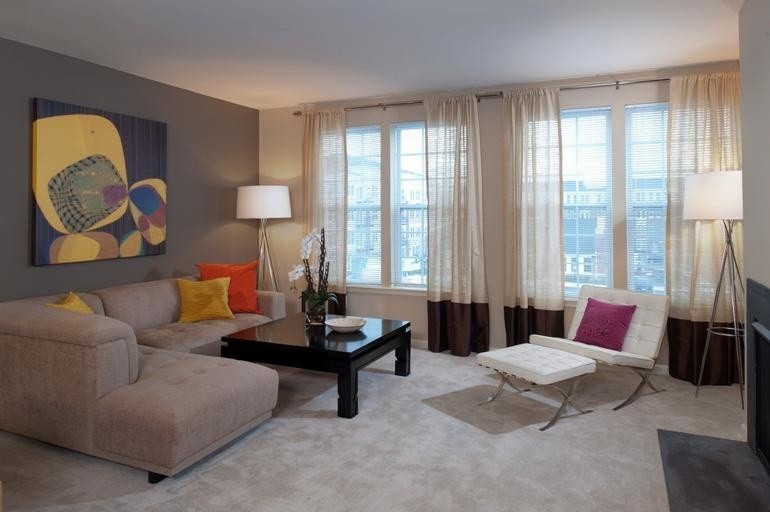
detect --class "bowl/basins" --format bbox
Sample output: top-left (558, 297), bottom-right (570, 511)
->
top-left (324, 317), bottom-right (367, 333)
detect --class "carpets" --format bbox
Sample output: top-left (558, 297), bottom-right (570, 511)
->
top-left (652, 424), bottom-right (770, 511)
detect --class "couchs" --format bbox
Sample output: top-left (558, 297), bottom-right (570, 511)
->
top-left (0, 272), bottom-right (289, 484)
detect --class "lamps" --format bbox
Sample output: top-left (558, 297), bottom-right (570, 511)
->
top-left (236, 183), bottom-right (293, 292)
top-left (677, 168), bottom-right (743, 409)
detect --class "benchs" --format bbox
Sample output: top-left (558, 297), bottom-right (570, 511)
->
top-left (528, 284), bottom-right (672, 411)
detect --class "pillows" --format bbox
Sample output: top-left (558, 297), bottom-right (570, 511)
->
top-left (197, 258), bottom-right (264, 317)
top-left (574, 295), bottom-right (637, 352)
top-left (175, 274), bottom-right (236, 324)
top-left (51, 293), bottom-right (94, 316)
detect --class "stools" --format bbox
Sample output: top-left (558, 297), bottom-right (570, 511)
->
top-left (476, 342), bottom-right (597, 432)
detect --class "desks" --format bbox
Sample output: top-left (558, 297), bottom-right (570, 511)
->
top-left (220, 310), bottom-right (411, 421)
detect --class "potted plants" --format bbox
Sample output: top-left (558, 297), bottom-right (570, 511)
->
top-left (298, 224), bottom-right (341, 325)
